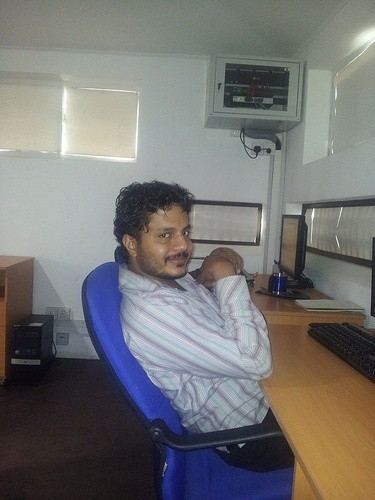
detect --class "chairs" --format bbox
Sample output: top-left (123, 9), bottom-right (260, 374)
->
top-left (81, 261), bottom-right (295, 500)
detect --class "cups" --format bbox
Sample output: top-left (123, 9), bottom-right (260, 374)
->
top-left (268, 272), bottom-right (288, 296)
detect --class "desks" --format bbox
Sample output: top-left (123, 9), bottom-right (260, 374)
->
top-left (249, 271), bottom-right (366, 324)
top-left (257, 323), bottom-right (375, 500)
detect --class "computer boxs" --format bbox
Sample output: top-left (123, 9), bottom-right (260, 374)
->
top-left (8, 314), bottom-right (54, 388)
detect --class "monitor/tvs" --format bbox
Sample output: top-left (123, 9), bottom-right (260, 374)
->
top-left (278, 214), bottom-right (308, 288)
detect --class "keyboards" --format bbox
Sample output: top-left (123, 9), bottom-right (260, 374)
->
top-left (307, 321), bottom-right (375, 383)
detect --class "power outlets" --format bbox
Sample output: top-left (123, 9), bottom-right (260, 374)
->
top-left (58, 307), bottom-right (70, 320)
top-left (251, 142), bottom-right (264, 155)
top-left (46, 307), bottom-right (58, 320)
top-left (263, 143), bottom-right (274, 155)
top-left (56, 333), bottom-right (69, 345)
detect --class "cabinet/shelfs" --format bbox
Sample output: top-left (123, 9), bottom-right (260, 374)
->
top-left (0, 256), bottom-right (35, 383)
